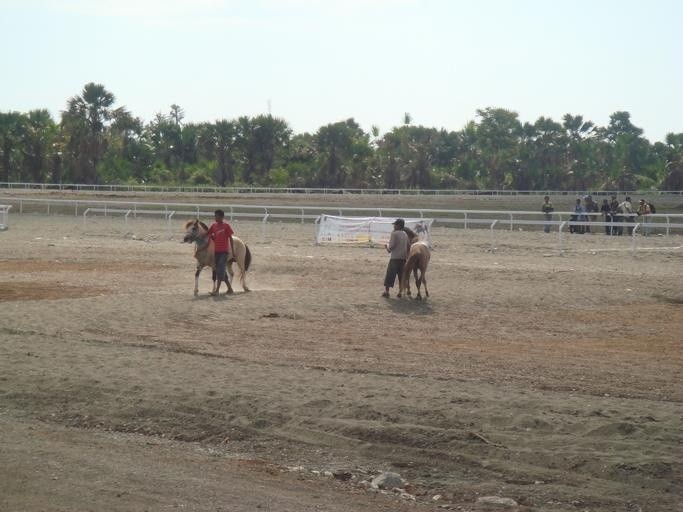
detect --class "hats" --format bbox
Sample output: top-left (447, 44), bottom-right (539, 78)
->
top-left (391, 219), bottom-right (404, 226)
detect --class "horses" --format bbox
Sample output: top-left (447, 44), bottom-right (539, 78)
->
top-left (183, 218), bottom-right (252, 297)
top-left (396, 226), bottom-right (431, 299)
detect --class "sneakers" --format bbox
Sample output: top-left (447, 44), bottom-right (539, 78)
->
top-left (226, 289), bottom-right (233, 295)
top-left (382, 292), bottom-right (390, 297)
top-left (397, 294), bottom-right (402, 298)
top-left (209, 291), bottom-right (219, 295)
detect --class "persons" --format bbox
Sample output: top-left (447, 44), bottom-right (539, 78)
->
top-left (608, 200), bottom-right (623, 235)
top-left (582, 194), bottom-right (598, 234)
top-left (541, 195), bottom-right (553, 233)
top-left (635, 198), bottom-right (654, 236)
top-left (600, 198), bottom-right (610, 234)
top-left (619, 196), bottom-right (634, 235)
top-left (379, 218), bottom-right (409, 300)
top-left (573, 198), bottom-right (585, 233)
top-left (199, 209), bottom-right (236, 296)
